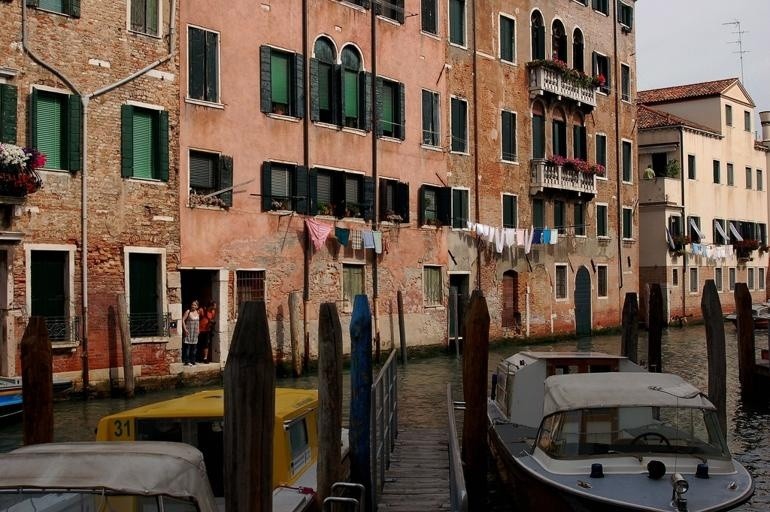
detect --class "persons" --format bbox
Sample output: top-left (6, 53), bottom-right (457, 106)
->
top-left (181, 299), bottom-right (201, 366)
top-left (196, 307), bottom-right (216, 364)
top-left (196, 300), bottom-right (218, 317)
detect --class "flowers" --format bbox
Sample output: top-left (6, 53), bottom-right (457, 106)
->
top-left (525, 56), bottom-right (607, 88)
top-left (545, 154), bottom-right (606, 177)
top-left (0, 142), bottom-right (48, 195)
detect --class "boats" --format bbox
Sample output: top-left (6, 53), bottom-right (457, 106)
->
top-left (0, 376), bottom-right (73, 419)
top-left (0, 386), bottom-right (350, 512)
top-left (487, 350), bottom-right (755, 512)
top-left (724, 302), bottom-right (770, 330)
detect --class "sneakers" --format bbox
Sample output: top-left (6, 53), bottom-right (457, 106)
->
top-left (186, 362), bottom-right (200, 366)
